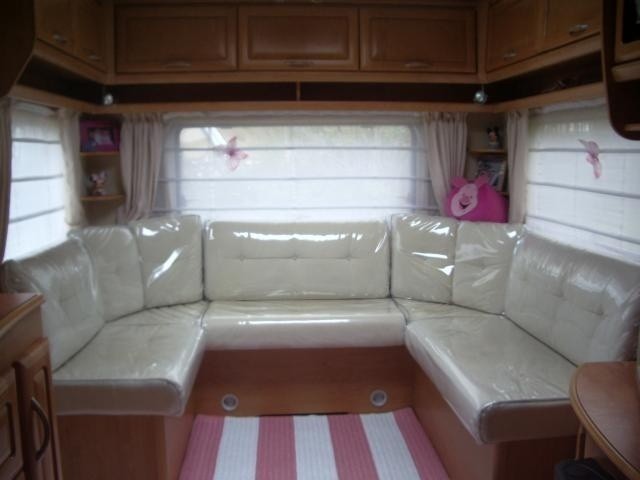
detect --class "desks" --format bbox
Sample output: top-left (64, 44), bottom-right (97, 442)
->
top-left (570, 361), bottom-right (639, 480)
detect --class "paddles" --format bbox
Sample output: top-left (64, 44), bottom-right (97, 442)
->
top-left (178, 406), bottom-right (453, 479)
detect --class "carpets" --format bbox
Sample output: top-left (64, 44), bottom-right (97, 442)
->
top-left (178, 406), bottom-right (453, 479)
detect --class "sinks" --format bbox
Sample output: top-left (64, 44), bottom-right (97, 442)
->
top-left (1, 214), bottom-right (638, 480)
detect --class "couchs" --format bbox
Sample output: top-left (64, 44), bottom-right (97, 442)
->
top-left (1, 214), bottom-right (638, 480)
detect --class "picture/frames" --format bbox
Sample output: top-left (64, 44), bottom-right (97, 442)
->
top-left (78, 119), bottom-right (121, 152)
top-left (570, 361), bottom-right (639, 480)
top-left (465, 156), bottom-right (507, 192)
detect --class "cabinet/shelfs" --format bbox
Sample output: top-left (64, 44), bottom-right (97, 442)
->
top-left (1, 293), bottom-right (65, 479)
top-left (1, 0), bottom-right (639, 139)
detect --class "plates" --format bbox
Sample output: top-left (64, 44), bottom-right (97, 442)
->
top-left (1, 293), bottom-right (65, 479)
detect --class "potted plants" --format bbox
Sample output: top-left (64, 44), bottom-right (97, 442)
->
top-left (465, 156), bottom-right (507, 192)
top-left (78, 119), bottom-right (121, 152)
